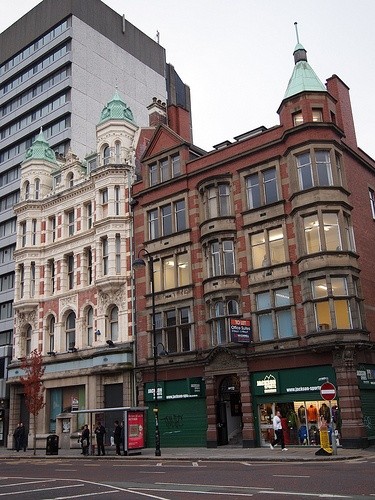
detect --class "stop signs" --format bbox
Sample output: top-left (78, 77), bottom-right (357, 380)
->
top-left (319, 382), bottom-right (336, 400)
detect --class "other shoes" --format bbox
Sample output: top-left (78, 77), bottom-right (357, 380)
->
top-left (80, 453), bottom-right (84, 455)
top-left (282, 448), bottom-right (288, 451)
top-left (96, 452), bottom-right (101, 456)
top-left (270, 444), bottom-right (274, 449)
top-left (84, 454), bottom-right (89, 456)
top-left (101, 453), bottom-right (105, 456)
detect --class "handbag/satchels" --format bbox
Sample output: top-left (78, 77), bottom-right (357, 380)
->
top-left (83, 440), bottom-right (87, 447)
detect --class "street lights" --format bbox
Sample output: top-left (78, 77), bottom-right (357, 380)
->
top-left (132, 248), bottom-right (169, 457)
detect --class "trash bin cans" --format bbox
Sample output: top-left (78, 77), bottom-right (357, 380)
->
top-left (46, 435), bottom-right (59, 455)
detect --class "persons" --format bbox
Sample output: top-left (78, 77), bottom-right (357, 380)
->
top-left (111, 419), bottom-right (123, 456)
top-left (95, 422), bottom-right (108, 456)
top-left (13, 424), bottom-right (28, 453)
top-left (270, 410), bottom-right (289, 453)
top-left (81, 424), bottom-right (91, 456)
